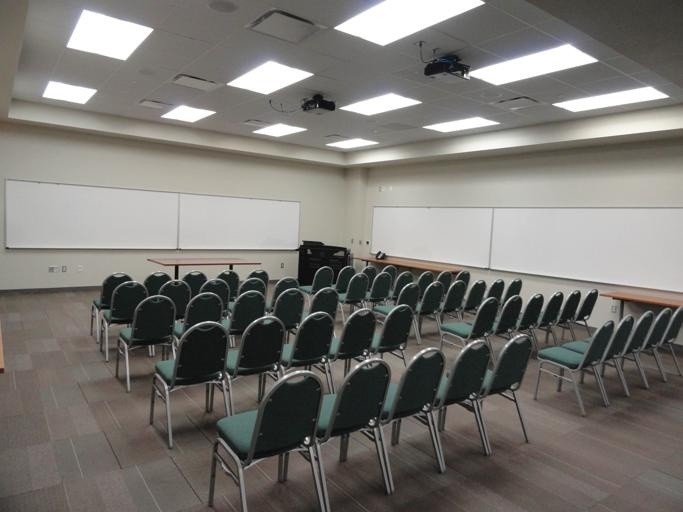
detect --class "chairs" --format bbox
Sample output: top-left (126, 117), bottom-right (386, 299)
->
top-left (100, 281), bottom-right (152, 362)
top-left (380, 264), bottom-right (398, 288)
top-left (89, 271), bottom-right (134, 344)
top-left (207, 369), bottom-right (326, 512)
top-left (281, 311), bottom-right (334, 395)
top-left (272, 288), bottom-right (306, 343)
top-left (283, 358), bottom-right (394, 512)
top-left (240, 277), bottom-right (266, 296)
top-left (559, 314), bottom-right (634, 408)
top-left (210, 316), bottom-right (285, 423)
top-left (454, 270), bottom-right (470, 290)
top-left (479, 279), bottom-right (504, 306)
top-left (388, 271), bottom-right (415, 306)
top-left (157, 280), bottom-right (191, 321)
top-left (621, 307), bottom-right (672, 390)
top-left (443, 333), bottom-right (532, 458)
top-left (199, 278), bottom-right (230, 315)
top-left (582, 310), bottom-right (655, 397)
top-left (308, 287), bottom-right (339, 321)
top-left (415, 281), bottom-right (443, 343)
top-left (218, 270), bottom-right (239, 296)
top-left (435, 269), bottom-right (453, 295)
top-left (246, 269), bottom-right (269, 290)
top-left (550, 290), bottom-right (577, 347)
top-left (533, 320), bottom-right (615, 417)
top-left (503, 278), bottom-right (522, 307)
top-left (371, 303), bottom-right (415, 368)
top-left (368, 272), bottom-right (392, 311)
top-left (150, 320), bottom-right (232, 449)
top-left (344, 348), bottom-right (446, 496)
top-left (564, 289), bottom-right (599, 342)
top-left (415, 271), bottom-right (435, 301)
top-left (397, 341), bottom-right (490, 474)
top-left (266, 277), bottom-right (299, 316)
top-left (534, 291), bottom-right (564, 354)
top-left (336, 265), bottom-right (356, 294)
top-left (143, 271), bottom-right (182, 357)
top-left (374, 283), bottom-right (421, 346)
top-left (114, 294), bottom-right (177, 392)
top-left (182, 271), bottom-right (207, 290)
top-left (466, 295), bottom-right (523, 371)
top-left (226, 291), bottom-right (266, 347)
top-left (653, 305), bottom-right (683, 383)
top-left (336, 273), bottom-right (370, 327)
top-left (494, 294), bottom-right (544, 360)
top-left (361, 266), bottom-right (377, 285)
top-left (461, 280), bottom-right (486, 320)
top-left (298, 265), bottom-right (334, 314)
top-left (438, 280), bottom-right (464, 338)
top-left (440, 296), bottom-right (497, 373)
top-left (170, 292), bottom-right (223, 359)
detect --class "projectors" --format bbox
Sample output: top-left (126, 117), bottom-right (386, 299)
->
top-left (424, 60), bottom-right (470, 83)
top-left (301, 99), bottom-right (336, 116)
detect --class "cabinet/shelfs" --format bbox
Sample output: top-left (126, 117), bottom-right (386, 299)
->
top-left (298, 241), bottom-right (349, 285)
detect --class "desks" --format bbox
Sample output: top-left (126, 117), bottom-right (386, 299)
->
top-left (600, 285), bottom-right (682, 322)
top-left (148, 256), bottom-right (261, 281)
top-left (359, 254), bottom-right (466, 274)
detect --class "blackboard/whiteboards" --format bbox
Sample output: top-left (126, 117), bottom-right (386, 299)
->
top-left (4, 177), bottom-right (300, 251)
top-left (370, 208), bottom-right (683, 292)
